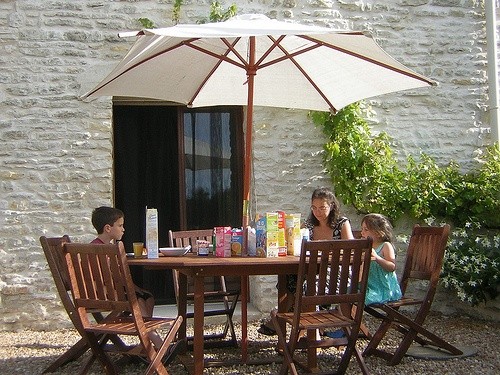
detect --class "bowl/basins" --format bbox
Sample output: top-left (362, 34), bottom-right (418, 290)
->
top-left (159, 248), bottom-right (189, 256)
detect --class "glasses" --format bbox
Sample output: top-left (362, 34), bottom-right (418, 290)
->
top-left (310, 205), bottom-right (331, 212)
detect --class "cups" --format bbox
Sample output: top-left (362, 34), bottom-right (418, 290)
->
top-left (133, 242), bottom-right (144, 258)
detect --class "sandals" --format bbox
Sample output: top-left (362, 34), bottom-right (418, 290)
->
top-left (258, 326), bottom-right (277, 335)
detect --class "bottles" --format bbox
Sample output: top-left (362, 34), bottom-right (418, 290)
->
top-left (196, 240), bottom-right (213, 256)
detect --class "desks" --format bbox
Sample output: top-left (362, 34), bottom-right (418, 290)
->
top-left (129, 256), bottom-right (364, 371)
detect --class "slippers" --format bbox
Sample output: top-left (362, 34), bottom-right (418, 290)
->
top-left (162, 338), bottom-right (185, 367)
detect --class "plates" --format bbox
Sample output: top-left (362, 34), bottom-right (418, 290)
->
top-left (126, 253), bottom-right (147, 256)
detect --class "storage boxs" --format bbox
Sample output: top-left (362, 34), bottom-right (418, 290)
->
top-left (246, 212), bottom-right (302, 259)
top-left (231, 228), bottom-right (241, 256)
top-left (213, 227), bottom-right (231, 258)
top-left (145, 206), bottom-right (159, 258)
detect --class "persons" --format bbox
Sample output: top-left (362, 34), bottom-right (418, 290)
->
top-left (325, 213), bottom-right (402, 338)
top-left (69, 206), bottom-right (178, 368)
top-left (257, 188), bottom-right (355, 350)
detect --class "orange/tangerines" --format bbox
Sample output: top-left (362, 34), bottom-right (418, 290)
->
top-left (232, 242), bottom-right (241, 251)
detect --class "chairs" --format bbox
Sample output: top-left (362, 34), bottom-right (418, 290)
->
top-left (36, 234), bottom-right (130, 375)
top-left (62, 242), bottom-right (184, 375)
top-left (271, 237), bottom-right (372, 375)
top-left (360, 225), bottom-right (463, 365)
top-left (167, 230), bottom-right (241, 351)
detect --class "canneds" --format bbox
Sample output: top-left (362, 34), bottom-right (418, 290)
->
top-left (197, 241), bottom-right (209, 256)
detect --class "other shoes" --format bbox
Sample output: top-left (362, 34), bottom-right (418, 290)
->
top-left (327, 330), bottom-right (344, 337)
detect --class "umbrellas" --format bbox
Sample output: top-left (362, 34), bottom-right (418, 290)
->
top-left (78, 14), bottom-right (440, 360)
